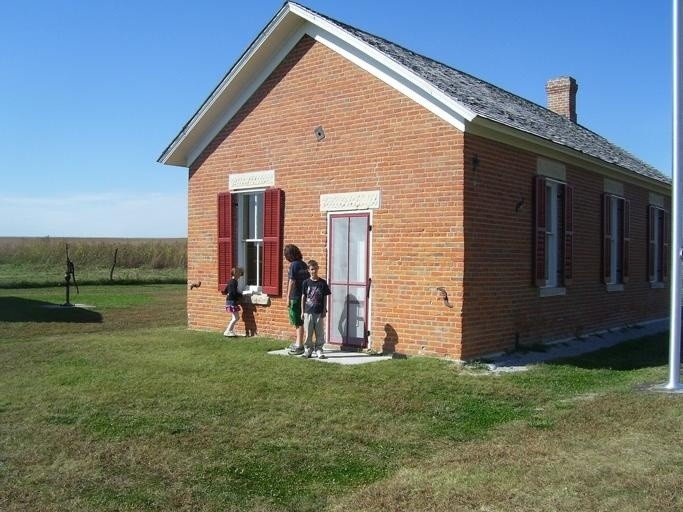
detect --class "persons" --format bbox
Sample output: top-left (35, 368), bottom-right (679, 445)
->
top-left (300, 259), bottom-right (331, 360)
top-left (223, 266), bottom-right (255, 337)
top-left (282, 243), bottom-right (310, 356)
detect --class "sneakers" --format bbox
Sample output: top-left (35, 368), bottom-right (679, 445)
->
top-left (315, 350), bottom-right (325, 359)
top-left (224, 331), bottom-right (235, 337)
top-left (285, 344), bottom-right (313, 358)
